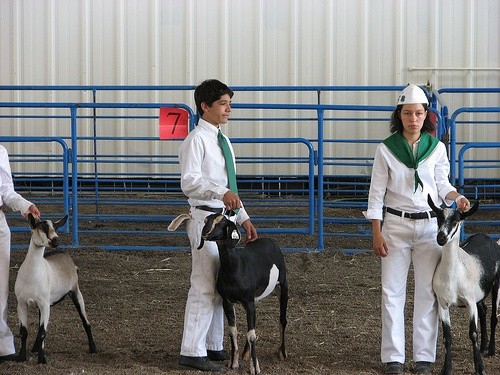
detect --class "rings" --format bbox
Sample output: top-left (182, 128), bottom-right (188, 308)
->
top-left (230, 206), bottom-right (233, 208)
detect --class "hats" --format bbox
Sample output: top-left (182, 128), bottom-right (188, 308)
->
top-left (397, 84), bottom-right (429, 106)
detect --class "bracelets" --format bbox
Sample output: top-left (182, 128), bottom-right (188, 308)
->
top-left (455, 195), bottom-right (465, 203)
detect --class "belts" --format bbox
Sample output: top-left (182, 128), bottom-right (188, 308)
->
top-left (383, 206), bottom-right (436, 220)
top-left (193, 205), bottom-right (236, 216)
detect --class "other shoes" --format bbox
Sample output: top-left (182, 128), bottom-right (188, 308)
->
top-left (179, 355), bottom-right (223, 371)
top-left (207, 349), bottom-right (231, 361)
top-left (385, 362), bottom-right (403, 374)
top-left (415, 361), bottom-right (431, 374)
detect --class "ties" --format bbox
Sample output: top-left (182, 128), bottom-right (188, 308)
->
top-left (218, 129), bottom-right (240, 215)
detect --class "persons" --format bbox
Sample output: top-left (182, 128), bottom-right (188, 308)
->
top-left (0, 144), bottom-right (40, 360)
top-left (362, 85), bottom-right (470, 375)
top-left (179, 79), bottom-right (257, 371)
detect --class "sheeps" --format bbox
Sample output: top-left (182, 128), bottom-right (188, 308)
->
top-left (427, 193), bottom-right (500, 375)
top-left (14, 213), bottom-right (96, 364)
top-left (203, 213), bottom-right (289, 375)
